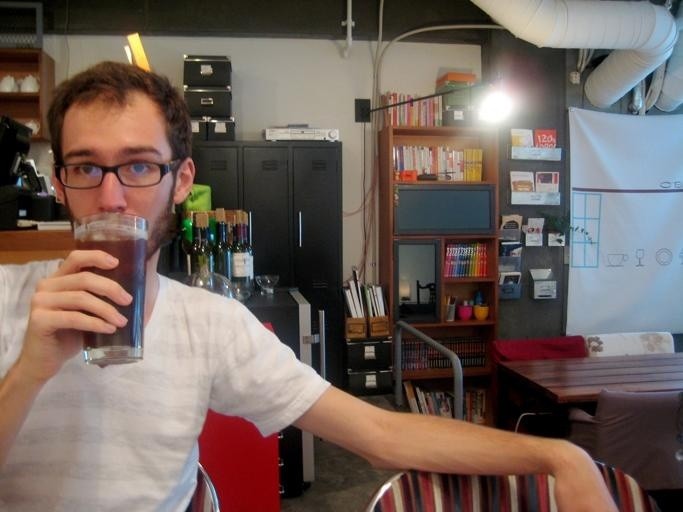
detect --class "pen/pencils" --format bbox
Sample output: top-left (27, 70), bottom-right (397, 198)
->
top-left (447, 295), bottom-right (458, 305)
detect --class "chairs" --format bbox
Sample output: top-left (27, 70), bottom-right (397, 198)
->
top-left (489, 331), bottom-right (683, 492)
top-left (364, 458), bottom-right (662, 512)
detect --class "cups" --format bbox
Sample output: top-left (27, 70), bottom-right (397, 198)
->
top-left (457, 304), bottom-right (473, 321)
top-left (72, 213), bottom-right (148, 366)
top-left (445, 304), bottom-right (456, 322)
top-left (473, 304), bottom-right (489, 320)
top-left (24, 120), bottom-right (40, 135)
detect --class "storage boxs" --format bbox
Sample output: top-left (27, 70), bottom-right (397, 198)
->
top-left (345, 336), bottom-right (393, 397)
top-left (182, 54), bottom-right (236, 141)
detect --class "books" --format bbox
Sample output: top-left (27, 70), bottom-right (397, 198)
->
top-left (499, 240), bottom-right (522, 266)
top-left (342, 265), bottom-right (386, 319)
top-left (379, 91), bottom-right (443, 127)
top-left (500, 215), bottom-right (523, 232)
top-left (399, 334), bottom-right (489, 370)
top-left (533, 128), bottom-right (557, 148)
top-left (443, 240), bottom-right (491, 278)
top-left (535, 172), bottom-right (559, 194)
top-left (499, 271), bottom-right (520, 287)
top-left (392, 142), bottom-right (483, 182)
top-left (510, 129), bottom-right (532, 148)
top-left (510, 170), bottom-right (534, 195)
top-left (401, 378), bottom-right (493, 422)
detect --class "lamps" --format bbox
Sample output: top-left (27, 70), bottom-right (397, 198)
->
top-left (355, 71), bottom-right (512, 122)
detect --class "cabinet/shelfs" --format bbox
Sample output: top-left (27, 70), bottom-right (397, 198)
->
top-left (379, 126), bottom-right (499, 398)
top-left (0, 48), bottom-right (56, 142)
top-left (192, 141), bottom-right (342, 511)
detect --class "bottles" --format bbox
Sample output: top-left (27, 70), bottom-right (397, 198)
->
top-left (181, 207), bottom-right (254, 292)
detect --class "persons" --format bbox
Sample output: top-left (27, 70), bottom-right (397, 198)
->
top-left (0, 58), bottom-right (620, 512)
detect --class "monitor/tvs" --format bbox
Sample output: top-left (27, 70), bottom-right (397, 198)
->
top-left (0, 115), bottom-right (31, 188)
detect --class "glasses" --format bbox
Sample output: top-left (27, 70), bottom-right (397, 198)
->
top-left (54, 153), bottom-right (182, 189)
